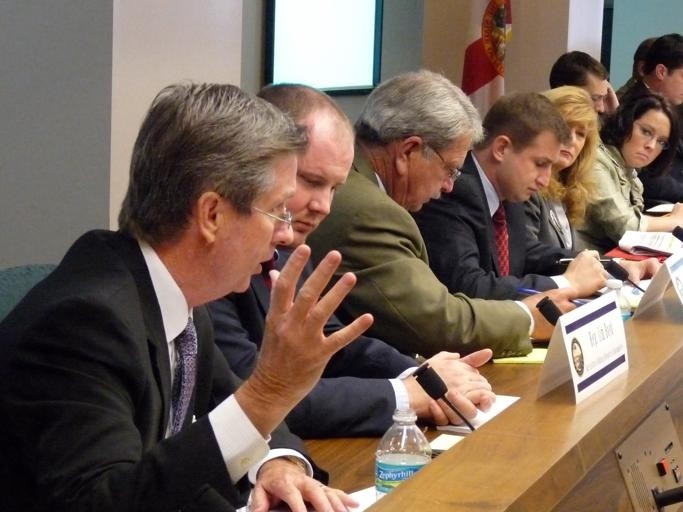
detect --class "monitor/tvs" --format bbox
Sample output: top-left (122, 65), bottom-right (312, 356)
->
top-left (264, 1), bottom-right (384, 101)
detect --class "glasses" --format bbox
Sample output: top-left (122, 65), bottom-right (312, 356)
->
top-left (251, 205), bottom-right (291, 230)
top-left (430, 145), bottom-right (461, 180)
top-left (635, 121), bottom-right (669, 150)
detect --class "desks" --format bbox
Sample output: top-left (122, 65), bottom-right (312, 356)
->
top-left (239, 242), bottom-right (683, 511)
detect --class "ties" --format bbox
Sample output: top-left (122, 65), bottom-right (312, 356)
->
top-left (492, 203), bottom-right (510, 277)
top-left (168, 318), bottom-right (199, 437)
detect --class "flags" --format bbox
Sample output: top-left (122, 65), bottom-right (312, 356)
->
top-left (462, 1), bottom-right (511, 127)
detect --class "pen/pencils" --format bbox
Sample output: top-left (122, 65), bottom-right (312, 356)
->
top-left (555, 256), bottom-right (624, 265)
top-left (516, 288), bottom-right (586, 307)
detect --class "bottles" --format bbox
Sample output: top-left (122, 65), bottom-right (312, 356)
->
top-left (370, 406), bottom-right (436, 504)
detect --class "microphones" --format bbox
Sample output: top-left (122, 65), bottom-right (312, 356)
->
top-left (412, 364), bottom-right (475, 433)
top-left (533, 296), bottom-right (564, 326)
top-left (601, 259), bottom-right (646, 295)
top-left (670, 225), bottom-right (683, 243)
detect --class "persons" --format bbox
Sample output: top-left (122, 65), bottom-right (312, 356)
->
top-left (304, 66), bottom-right (578, 362)
top-left (597, 34), bottom-right (682, 206)
top-left (524, 83), bottom-right (661, 285)
top-left (202, 84), bottom-right (500, 439)
top-left (547, 51), bottom-right (620, 123)
top-left (590, 87), bottom-right (682, 232)
top-left (0, 84), bottom-right (373, 512)
top-left (410, 88), bottom-right (609, 302)
top-left (611, 36), bottom-right (657, 104)
